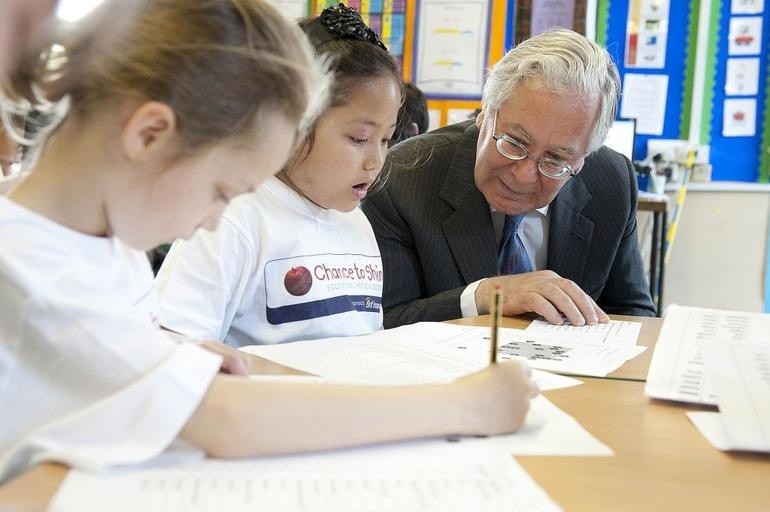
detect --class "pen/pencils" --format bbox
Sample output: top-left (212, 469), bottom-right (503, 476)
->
top-left (491, 285), bottom-right (501, 362)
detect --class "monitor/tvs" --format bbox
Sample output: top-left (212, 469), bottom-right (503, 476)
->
top-left (602, 117), bottom-right (637, 162)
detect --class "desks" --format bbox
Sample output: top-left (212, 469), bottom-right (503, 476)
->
top-left (631, 192), bottom-right (664, 317)
top-left (228, 306), bottom-right (770, 511)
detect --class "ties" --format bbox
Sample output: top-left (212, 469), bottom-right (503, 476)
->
top-left (496, 208), bottom-right (535, 276)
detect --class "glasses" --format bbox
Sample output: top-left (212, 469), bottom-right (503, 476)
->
top-left (490, 105), bottom-right (585, 184)
